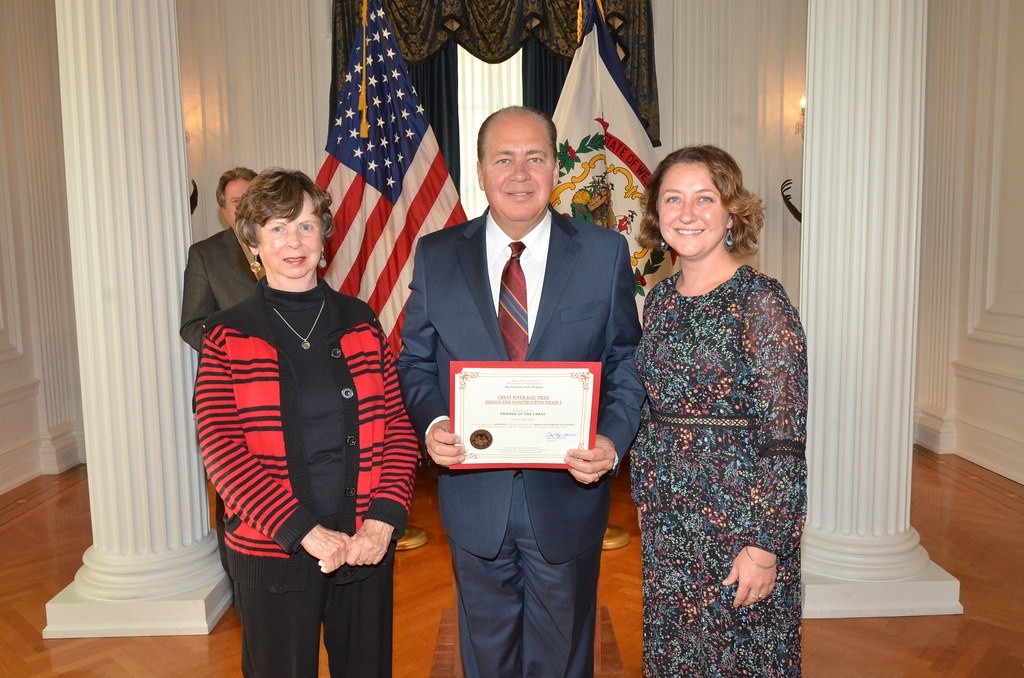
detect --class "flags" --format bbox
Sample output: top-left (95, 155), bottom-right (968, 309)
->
top-left (312, 0), bottom-right (468, 361)
top-left (550, 0), bottom-right (682, 330)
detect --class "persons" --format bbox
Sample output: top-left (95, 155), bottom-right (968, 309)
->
top-left (394, 106), bottom-right (648, 678)
top-left (629, 145), bottom-right (809, 678)
top-left (195, 167), bottom-right (420, 678)
top-left (181, 168), bottom-right (266, 592)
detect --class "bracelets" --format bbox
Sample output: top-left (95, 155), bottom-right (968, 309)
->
top-left (746, 546), bottom-right (778, 569)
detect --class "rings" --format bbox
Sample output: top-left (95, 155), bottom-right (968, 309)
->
top-left (595, 472), bottom-right (600, 483)
top-left (749, 591), bottom-right (763, 600)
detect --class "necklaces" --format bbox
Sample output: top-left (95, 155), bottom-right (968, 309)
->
top-left (273, 299), bottom-right (325, 350)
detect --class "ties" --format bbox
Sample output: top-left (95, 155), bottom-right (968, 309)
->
top-left (499, 241), bottom-right (528, 361)
